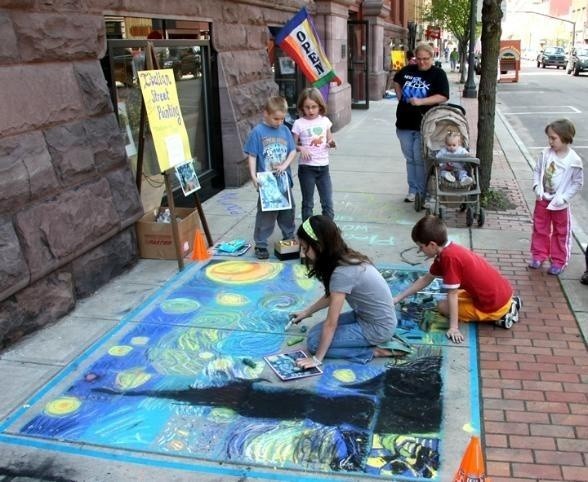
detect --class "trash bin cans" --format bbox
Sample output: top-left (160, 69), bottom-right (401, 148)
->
top-left (435, 61), bottom-right (441, 69)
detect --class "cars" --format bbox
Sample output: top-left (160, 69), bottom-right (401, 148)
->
top-left (535, 44), bottom-right (567, 69)
top-left (475, 53), bottom-right (481, 73)
top-left (161, 45), bottom-right (200, 82)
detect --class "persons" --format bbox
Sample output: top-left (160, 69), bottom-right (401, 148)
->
top-left (392, 217), bottom-right (522, 344)
top-left (527, 119), bottom-right (584, 276)
top-left (392, 43), bottom-right (450, 211)
top-left (240, 95), bottom-right (298, 259)
top-left (406, 46), bottom-right (460, 74)
top-left (288, 215), bottom-right (413, 371)
top-left (290, 87), bottom-right (337, 223)
top-left (435, 132), bottom-right (473, 187)
top-left (578, 244), bottom-right (588, 286)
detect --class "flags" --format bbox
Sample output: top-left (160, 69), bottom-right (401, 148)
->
top-left (274, 6), bottom-right (341, 105)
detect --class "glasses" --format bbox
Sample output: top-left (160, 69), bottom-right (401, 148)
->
top-left (416, 55), bottom-right (431, 60)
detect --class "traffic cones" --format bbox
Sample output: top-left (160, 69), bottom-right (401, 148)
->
top-left (448, 436), bottom-right (490, 481)
top-left (192, 229), bottom-right (211, 260)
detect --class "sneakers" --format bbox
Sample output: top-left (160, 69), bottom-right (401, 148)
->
top-left (460, 177), bottom-right (472, 186)
top-left (530, 258), bottom-right (543, 269)
top-left (405, 192), bottom-right (416, 202)
top-left (548, 265), bottom-right (565, 275)
top-left (254, 247), bottom-right (270, 259)
top-left (497, 295), bottom-right (521, 330)
top-left (445, 173), bottom-right (456, 182)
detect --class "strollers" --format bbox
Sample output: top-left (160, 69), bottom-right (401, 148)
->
top-left (411, 103), bottom-right (486, 228)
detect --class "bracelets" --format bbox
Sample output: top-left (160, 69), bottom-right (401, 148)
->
top-left (312, 355), bottom-right (323, 366)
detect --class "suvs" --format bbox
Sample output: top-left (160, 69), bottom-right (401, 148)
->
top-left (564, 43), bottom-right (588, 76)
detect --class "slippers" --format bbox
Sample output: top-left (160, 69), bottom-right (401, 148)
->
top-left (377, 332), bottom-right (412, 358)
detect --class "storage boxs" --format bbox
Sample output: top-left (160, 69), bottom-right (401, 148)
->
top-left (135, 205), bottom-right (201, 261)
